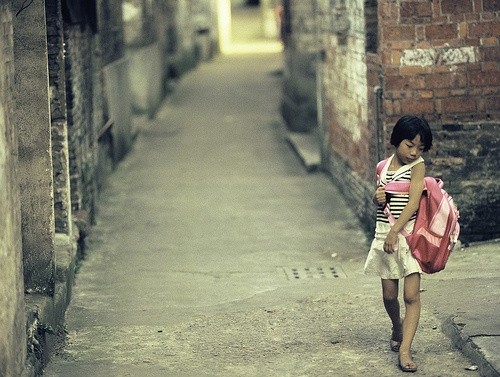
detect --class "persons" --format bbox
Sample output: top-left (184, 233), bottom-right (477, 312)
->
top-left (362, 113), bottom-right (433, 373)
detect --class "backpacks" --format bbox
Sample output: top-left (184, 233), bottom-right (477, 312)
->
top-left (376, 158), bottom-right (461, 274)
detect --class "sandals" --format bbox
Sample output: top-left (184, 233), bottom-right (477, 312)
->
top-left (390, 322), bottom-right (403, 351)
top-left (398, 351), bottom-right (418, 372)
